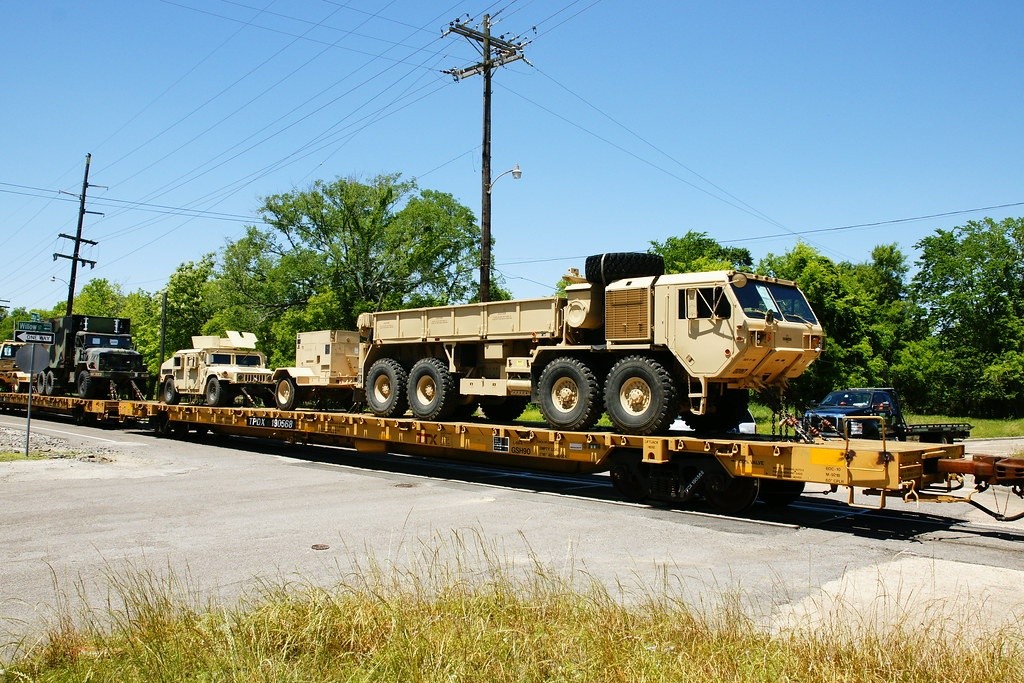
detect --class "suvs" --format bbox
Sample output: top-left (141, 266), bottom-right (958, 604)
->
top-left (160, 330), bottom-right (275, 406)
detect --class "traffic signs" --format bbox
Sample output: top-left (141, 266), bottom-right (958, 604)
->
top-left (13, 330), bottom-right (56, 346)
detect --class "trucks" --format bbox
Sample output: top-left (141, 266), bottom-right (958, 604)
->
top-left (36, 316), bottom-right (150, 398)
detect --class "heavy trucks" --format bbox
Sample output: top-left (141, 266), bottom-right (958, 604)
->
top-left (0, 339), bottom-right (966, 510)
top-left (275, 252), bottom-right (827, 431)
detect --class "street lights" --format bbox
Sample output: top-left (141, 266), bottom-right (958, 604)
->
top-left (481, 163), bottom-right (522, 303)
top-left (50, 275), bottom-right (75, 316)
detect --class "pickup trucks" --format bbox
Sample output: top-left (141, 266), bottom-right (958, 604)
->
top-left (802, 386), bottom-right (976, 445)
